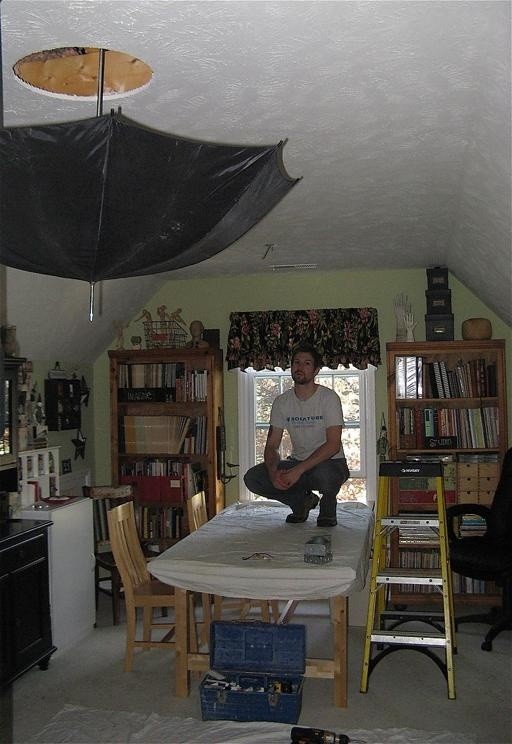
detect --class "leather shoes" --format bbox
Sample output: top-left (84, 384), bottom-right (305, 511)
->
top-left (317, 504), bottom-right (337, 527)
top-left (286, 495), bottom-right (319, 523)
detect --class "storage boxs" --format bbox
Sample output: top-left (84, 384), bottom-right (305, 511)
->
top-left (424, 266), bottom-right (454, 339)
top-left (198, 621), bottom-right (306, 724)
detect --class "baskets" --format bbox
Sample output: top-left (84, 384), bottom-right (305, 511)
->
top-left (143, 320), bottom-right (188, 351)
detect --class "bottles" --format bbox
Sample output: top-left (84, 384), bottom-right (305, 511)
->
top-left (55, 362), bottom-right (60, 370)
top-left (290, 727), bottom-right (350, 743)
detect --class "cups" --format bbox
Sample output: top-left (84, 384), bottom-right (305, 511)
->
top-left (18, 427), bottom-right (29, 450)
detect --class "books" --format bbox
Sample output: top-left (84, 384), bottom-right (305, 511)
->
top-left (118, 361), bottom-right (207, 540)
top-left (395, 355), bottom-right (505, 597)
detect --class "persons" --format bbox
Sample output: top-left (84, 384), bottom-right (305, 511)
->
top-left (184, 320), bottom-right (209, 349)
top-left (244, 345), bottom-right (349, 527)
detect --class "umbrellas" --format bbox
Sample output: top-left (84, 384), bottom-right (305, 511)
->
top-left (0, 46), bottom-right (303, 323)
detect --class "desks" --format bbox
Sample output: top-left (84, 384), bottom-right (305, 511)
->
top-left (145, 499), bottom-right (375, 708)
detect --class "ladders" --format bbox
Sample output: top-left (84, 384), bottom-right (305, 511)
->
top-left (358, 460), bottom-right (458, 701)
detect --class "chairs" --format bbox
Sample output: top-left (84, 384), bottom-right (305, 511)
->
top-left (104, 499), bottom-right (202, 675)
top-left (446, 446), bottom-right (512, 652)
top-left (187, 492), bottom-right (279, 626)
top-left (84, 485), bottom-right (170, 627)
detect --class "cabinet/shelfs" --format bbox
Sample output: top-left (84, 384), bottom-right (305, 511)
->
top-left (386, 339), bottom-right (506, 610)
top-left (0, 444), bottom-right (97, 697)
top-left (109, 349), bottom-right (225, 543)
